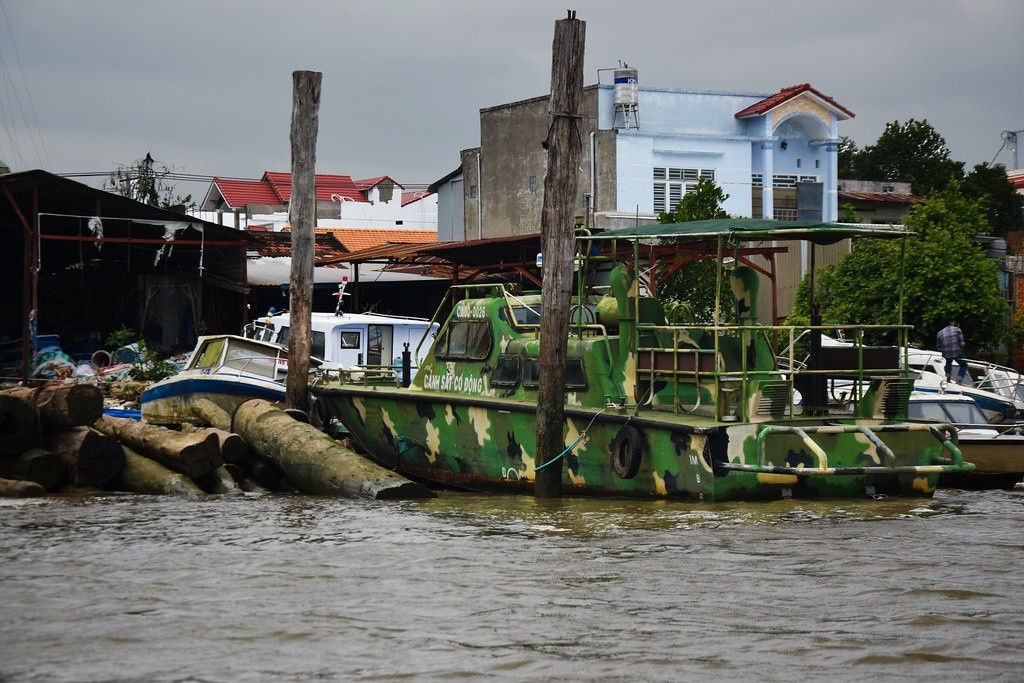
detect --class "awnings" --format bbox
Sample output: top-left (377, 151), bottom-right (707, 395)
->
top-left (314, 235), bottom-right (540, 264)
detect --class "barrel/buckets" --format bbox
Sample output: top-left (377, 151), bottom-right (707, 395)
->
top-left (91, 350), bottom-right (112, 369)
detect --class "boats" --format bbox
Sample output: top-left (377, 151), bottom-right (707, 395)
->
top-left (774, 330), bottom-right (1024, 488)
top-left (136, 334), bottom-right (331, 428)
top-left (306, 215), bottom-right (976, 501)
top-left (238, 273), bottom-right (443, 382)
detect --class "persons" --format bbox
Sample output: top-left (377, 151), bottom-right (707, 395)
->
top-left (937, 317), bottom-right (967, 384)
